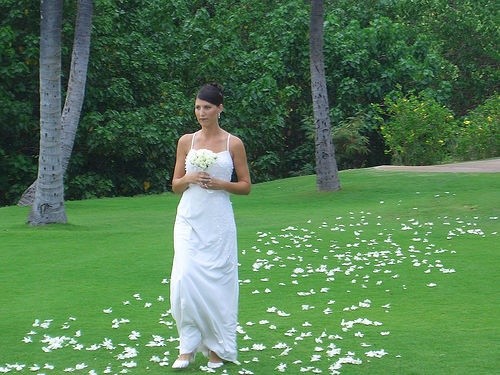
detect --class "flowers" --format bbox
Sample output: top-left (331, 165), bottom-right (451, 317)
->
top-left (189, 149), bottom-right (219, 171)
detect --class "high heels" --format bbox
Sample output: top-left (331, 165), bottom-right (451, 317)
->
top-left (207, 361), bottom-right (224, 368)
top-left (172, 352), bottom-right (195, 369)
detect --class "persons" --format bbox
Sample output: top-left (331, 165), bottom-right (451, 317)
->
top-left (169, 84), bottom-right (251, 369)
